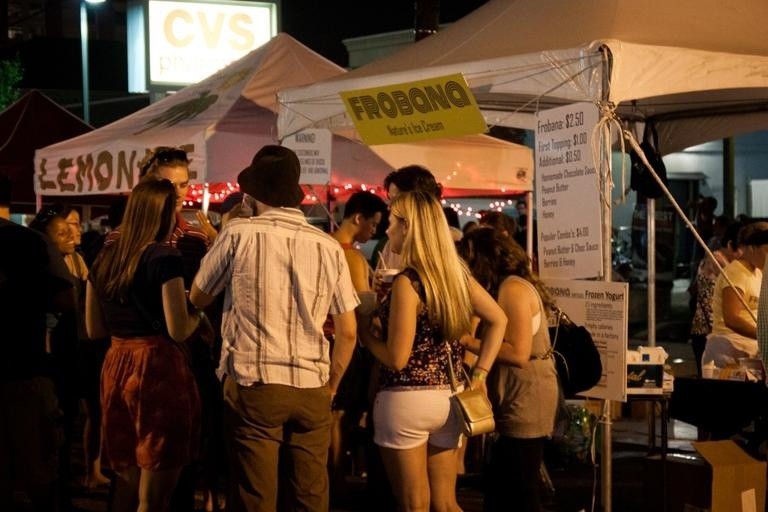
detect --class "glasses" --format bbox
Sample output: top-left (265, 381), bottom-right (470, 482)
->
top-left (141, 149), bottom-right (187, 175)
top-left (35, 208), bottom-right (62, 219)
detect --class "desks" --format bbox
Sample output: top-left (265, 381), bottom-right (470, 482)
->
top-left (620, 382), bottom-right (671, 459)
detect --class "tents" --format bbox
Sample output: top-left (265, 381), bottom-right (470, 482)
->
top-left (33, 36), bottom-right (535, 275)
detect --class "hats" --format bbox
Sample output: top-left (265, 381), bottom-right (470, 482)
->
top-left (237, 145), bottom-right (305, 208)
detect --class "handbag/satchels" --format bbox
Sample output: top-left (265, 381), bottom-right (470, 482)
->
top-left (630, 141), bottom-right (668, 199)
top-left (452, 390), bottom-right (497, 438)
top-left (548, 325), bottom-right (602, 396)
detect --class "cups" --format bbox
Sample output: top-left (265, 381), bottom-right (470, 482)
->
top-left (354, 289), bottom-right (377, 316)
top-left (379, 268), bottom-right (400, 307)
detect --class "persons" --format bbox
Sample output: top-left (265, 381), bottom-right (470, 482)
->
top-left (0, 143), bottom-right (561, 510)
top-left (686, 209), bottom-right (768, 382)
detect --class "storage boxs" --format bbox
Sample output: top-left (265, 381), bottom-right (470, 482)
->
top-left (641, 438), bottom-right (768, 512)
